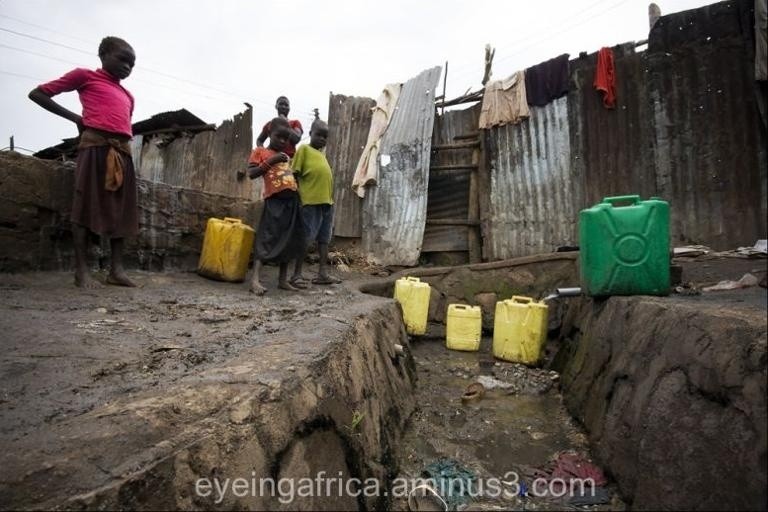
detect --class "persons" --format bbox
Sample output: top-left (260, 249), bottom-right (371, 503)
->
top-left (245, 118), bottom-right (301, 297)
top-left (290, 120), bottom-right (342, 289)
top-left (257, 96), bottom-right (304, 157)
top-left (27, 36), bottom-right (146, 291)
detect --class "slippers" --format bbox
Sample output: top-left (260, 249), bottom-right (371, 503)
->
top-left (291, 275), bottom-right (308, 288)
top-left (312, 275), bottom-right (341, 283)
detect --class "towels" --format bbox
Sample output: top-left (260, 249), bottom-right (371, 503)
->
top-left (592, 46), bottom-right (617, 111)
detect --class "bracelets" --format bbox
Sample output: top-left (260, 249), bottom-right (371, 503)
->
top-left (260, 160), bottom-right (271, 170)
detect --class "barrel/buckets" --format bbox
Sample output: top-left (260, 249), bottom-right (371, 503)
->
top-left (487, 293), bottom-right (551, 367)
top-left (577, 192), bottom-right (674, 298)
top-left (194, 215), bottom-right (256, 285)
top-left (392, 275), bottom-right (433, 336)
top-left (442, 302), bottom-right (483, 352)
